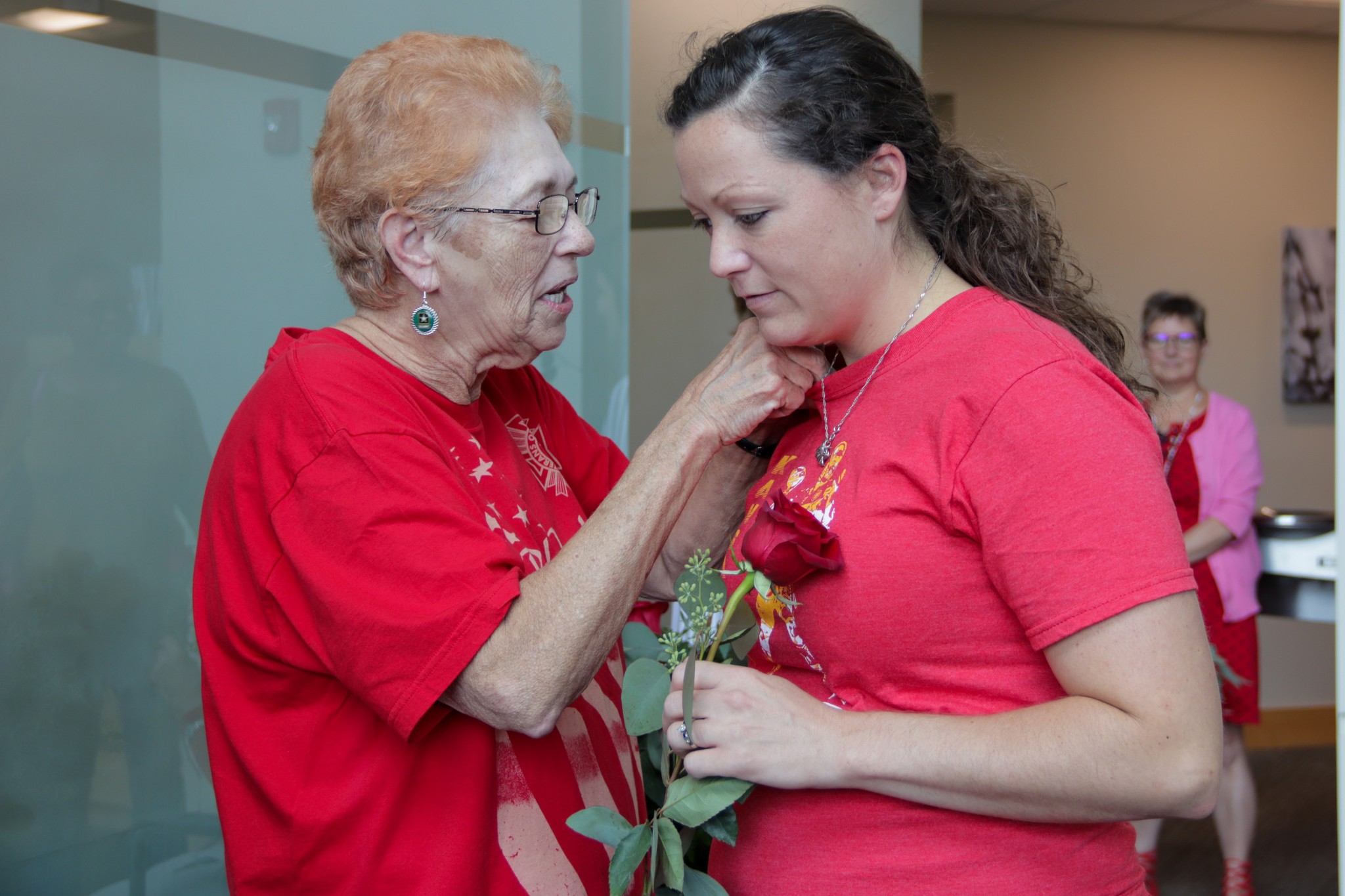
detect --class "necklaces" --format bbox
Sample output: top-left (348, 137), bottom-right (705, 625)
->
top-left (815, 253), bottom-right (942, 467)
top-left (1164, 390), bottom-right (1202, 478)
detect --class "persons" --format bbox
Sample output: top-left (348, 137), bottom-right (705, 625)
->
top-left (37, 263), bottom-right (211, 857)
top-left (1131, 291), bottom-right (1266, 896)
top-left (659, 4), bottom-right (1226, 896)
top-left (193, 31), bottom-right (835, 896)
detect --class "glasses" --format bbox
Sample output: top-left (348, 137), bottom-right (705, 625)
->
top-left (1147, 331), bottom-right (1199, 343)
top-left (418, 187), bottom-right (602, 237)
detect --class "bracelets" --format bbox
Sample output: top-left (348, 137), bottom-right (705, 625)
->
top-left (735, 438), bottom-right (775, 460)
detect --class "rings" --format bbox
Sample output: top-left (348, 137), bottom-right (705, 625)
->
top-left (677, 719), bottom-right (698, 751)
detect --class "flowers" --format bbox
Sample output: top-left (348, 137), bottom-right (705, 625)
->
top-left (565, 483), bottom-right (845, 896)
